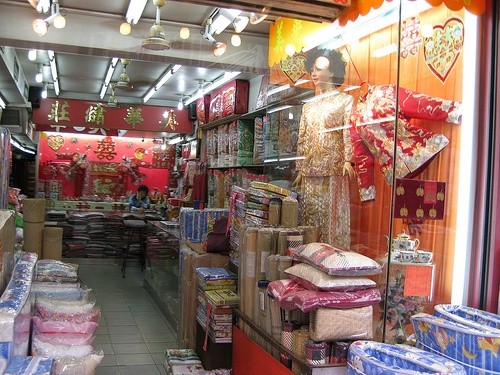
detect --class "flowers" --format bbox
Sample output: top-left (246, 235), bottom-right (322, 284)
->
top-left (379, 287), bottom-right (422, 343)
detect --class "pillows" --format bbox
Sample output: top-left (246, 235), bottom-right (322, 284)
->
top-left (264, 240), bottom-right (382, 310)
top-left (30, 258), bottom-right (105, 375)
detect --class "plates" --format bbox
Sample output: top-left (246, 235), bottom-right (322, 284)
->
top-left (415, 263), bottom-right (433, 265)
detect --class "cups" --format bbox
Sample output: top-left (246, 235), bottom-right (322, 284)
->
top-left (418, 252), bottom-right (432, 263)
top-left (400, 252), bottom-right (414, 263)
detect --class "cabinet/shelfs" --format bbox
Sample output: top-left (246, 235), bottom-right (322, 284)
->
top-left (146, 221), bottom-right (181, 332)
top-left (202, 90), bottom-right (314, 167)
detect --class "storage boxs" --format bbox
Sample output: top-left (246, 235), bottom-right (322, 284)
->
top-left (195, 78), bottom-right (252, 126)
top-left (196, 320), bottom-right (233, 371)
top-left (0, 209), bottom-right (16, 293)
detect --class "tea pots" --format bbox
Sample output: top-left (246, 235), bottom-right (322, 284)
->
top-left (383, 229), bottom-right (420, 261)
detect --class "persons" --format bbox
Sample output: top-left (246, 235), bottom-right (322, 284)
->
top-left (129, 185), bottom-right (150, 212)
top-left (293, 49), bottom-right (358, 248)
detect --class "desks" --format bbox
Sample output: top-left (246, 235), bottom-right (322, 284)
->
top-left (231, 308), bottom-right (346, 375)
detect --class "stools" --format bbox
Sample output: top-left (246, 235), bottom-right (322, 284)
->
top-left (120, 220), bottom-right (151, 276)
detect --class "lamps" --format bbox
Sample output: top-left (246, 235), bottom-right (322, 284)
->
top-left (19, 0), bottom-right (268, 106)
